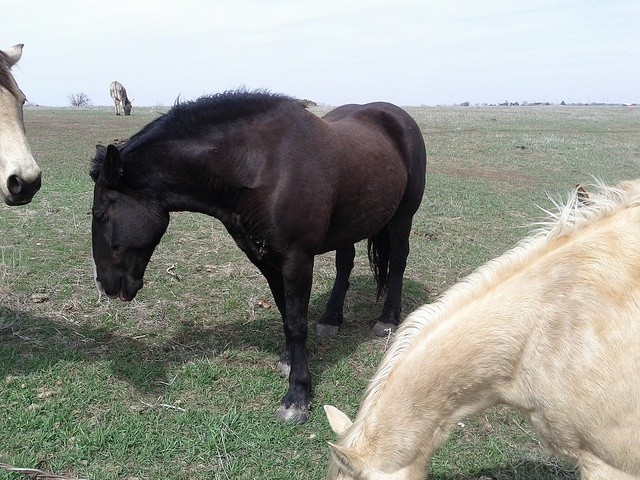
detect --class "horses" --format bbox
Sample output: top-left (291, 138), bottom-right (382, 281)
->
top-left (109, 79), bottom-right (133, 116)
top-left (321, 170), bottom-right (640, 480)
top-left (88, 86), bottom-right (427, 428)
top-left (0, 42), bottom-right (42, 206)
